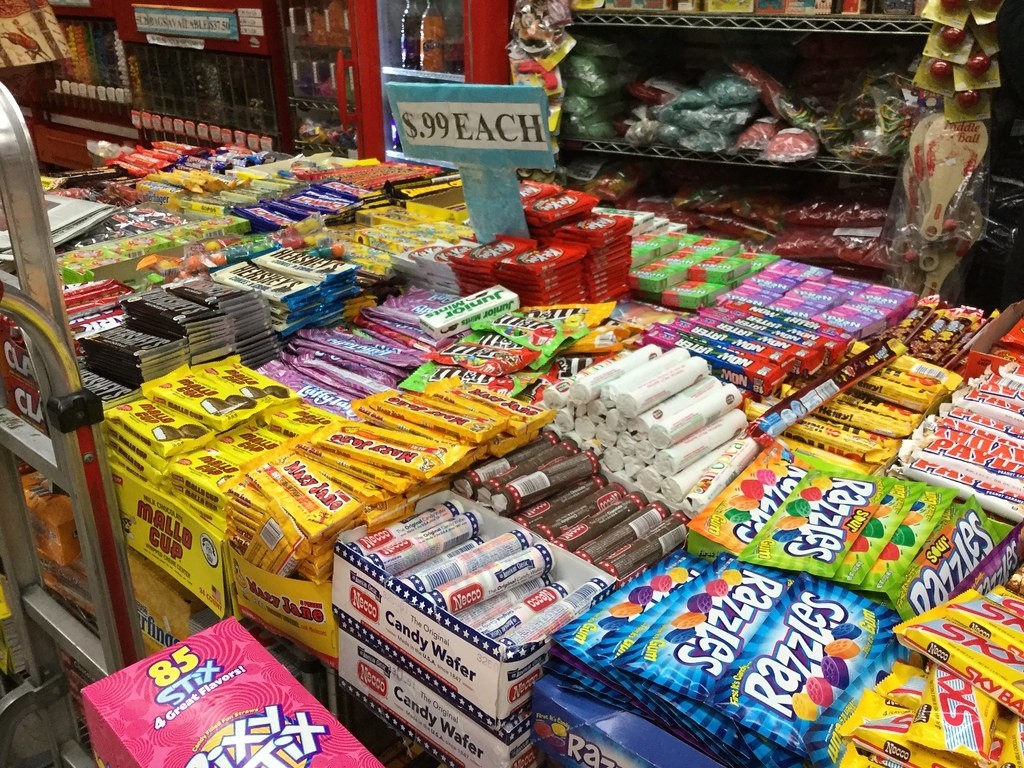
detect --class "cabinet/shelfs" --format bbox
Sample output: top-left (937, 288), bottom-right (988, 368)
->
top-left (501, 0), bottom-right (1024, 311)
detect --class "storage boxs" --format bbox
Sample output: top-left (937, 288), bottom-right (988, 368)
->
top-left (226, 489), bottom-right (620, 768)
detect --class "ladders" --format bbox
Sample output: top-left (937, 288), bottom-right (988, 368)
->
top-left (0, 82), bottom-right (149, 768)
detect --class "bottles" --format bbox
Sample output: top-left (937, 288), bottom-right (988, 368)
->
top-left (444, 0), bottom-right (464, 74)
top-left (401, 0), bottom-right (421, 70)
top-left (420, 0), bottom-right (445, 72)
